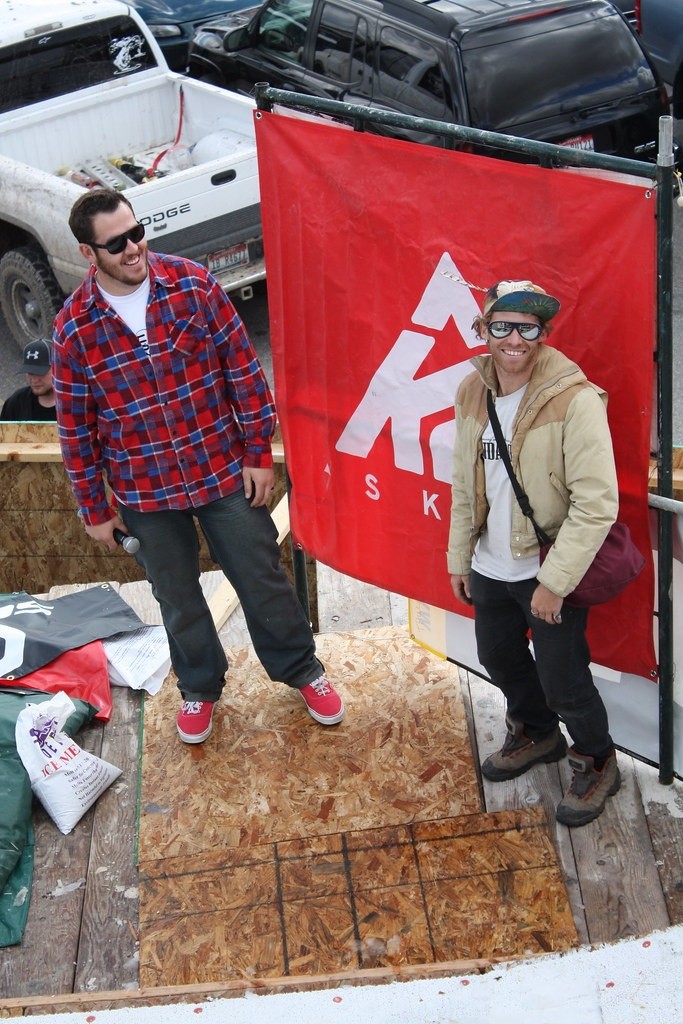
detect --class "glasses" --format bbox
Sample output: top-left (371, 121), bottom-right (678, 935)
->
top-left (88, 221), bottom-right (146, 255)
top-left (485, 321), bottom-right (542, 341)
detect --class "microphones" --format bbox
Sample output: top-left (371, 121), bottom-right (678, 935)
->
top-left (76, 510), bottom-right (140, 553)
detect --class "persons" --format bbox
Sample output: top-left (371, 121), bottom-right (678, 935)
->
top-left (447, 280), bottom-right (621, 826)
top-left (50, 189), bottom-right (343, 743)
top-left (0, 339), bottom-right (57, 421)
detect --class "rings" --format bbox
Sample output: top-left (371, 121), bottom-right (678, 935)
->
top-left (270, 487), bottom-right (274, 491)
top-left (531, 611), bottom-right (538, 615)
top-left (553, 616), bottom-right (561, 620)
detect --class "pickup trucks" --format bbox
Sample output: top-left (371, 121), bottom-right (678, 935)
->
top-left (0, 0), bottom-right (356, 354)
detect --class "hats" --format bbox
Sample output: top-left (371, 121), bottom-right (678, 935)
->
top-left (483, 280), bottom-right (562, 322)
top-left (16, 337), bottom-right (52, 375)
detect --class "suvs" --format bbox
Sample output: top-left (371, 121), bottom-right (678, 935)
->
top-left (128, 0), bottom-right (683, 176)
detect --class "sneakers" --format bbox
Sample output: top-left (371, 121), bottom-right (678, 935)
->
top-left (176, 701), bottom-right (216, 743)
top-left (555, 743), bottom-right (621, 827)
top-left (481, 714), bottom-right (568, 782)
top-left (296, 672), bottom-right (344, 725)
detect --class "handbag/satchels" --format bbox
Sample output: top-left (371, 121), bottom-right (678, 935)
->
top-left (539, 523), bottom-right (647, 607)
